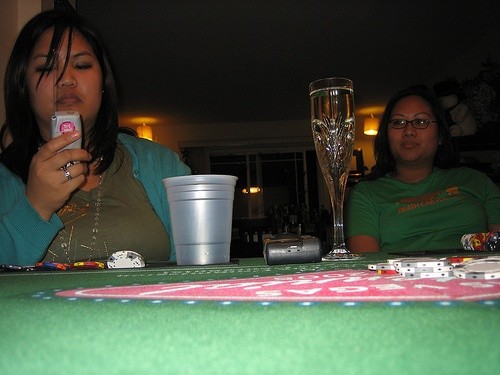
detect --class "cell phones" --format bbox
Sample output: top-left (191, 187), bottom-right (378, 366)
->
top-left (51, 111), bottom-right (81, 167)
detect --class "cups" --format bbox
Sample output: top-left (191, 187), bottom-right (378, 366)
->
top-left (162, 174), bottom-right (239, 266)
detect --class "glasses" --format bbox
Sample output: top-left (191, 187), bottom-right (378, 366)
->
top-left (388, 118), bottom-right (437, 129)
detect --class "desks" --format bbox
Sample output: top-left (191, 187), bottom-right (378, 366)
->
top-left (0, 252), bottom-right (500, 375)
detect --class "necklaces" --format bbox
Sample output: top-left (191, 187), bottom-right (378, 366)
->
top-left (59, 171), bottom-right (104, 263)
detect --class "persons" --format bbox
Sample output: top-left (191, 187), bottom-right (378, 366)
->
top-left (344, 85), bottom-right (500, 254)
top-left (0, 9), bottom-right (192, 265)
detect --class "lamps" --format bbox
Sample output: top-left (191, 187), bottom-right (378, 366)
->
top-left (136, 123), bottom-right (153, 141)
top-left (363, 114), bottom-right (380, 136)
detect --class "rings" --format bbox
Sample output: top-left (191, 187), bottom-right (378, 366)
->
top-left (63, 168), bottom-right (72, 180)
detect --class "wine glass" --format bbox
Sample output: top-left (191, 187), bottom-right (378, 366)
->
top-left (309, 77), bottom-right (364, 261)
